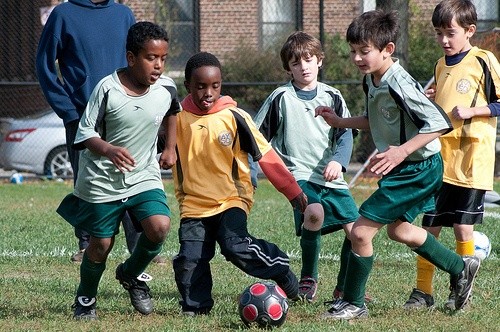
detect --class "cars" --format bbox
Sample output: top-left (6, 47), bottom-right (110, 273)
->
top-left (0, 110), bottom-right (173, 178)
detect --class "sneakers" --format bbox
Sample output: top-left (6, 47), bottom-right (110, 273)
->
top-left (297, 276), bottom-right (319, 302)
top-left (116, 261), bottom-right (154, 315)
top-left (182, 307), bottom-right (197, 317)
top-left (321, 299), bottom-right (369, 320)
top-left (403, 288), bottom-right (434, 309)
top-left (273, 268), bottom-right (298, 299)
top-left (450, 257), bottom-right (481, 310)
top-left (71, 288), bottom-right (97, 322)
top-left (444, 289), bottom-right (456, 312)
top-left (71, 249), bottom-right (86, 262)
top-left (151, 253), bottom-right (167, 265)
top-left (332, 289), bottom-right (371, 302)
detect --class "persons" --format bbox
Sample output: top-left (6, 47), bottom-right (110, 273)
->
top-left (247, 31), bottom-right (373, 304)
top-left (171, 52), bottom-right (308, 316)
top-left (314, 9), bottom-right (481, 321)
top-left (56, 21), bottom-right (183, 322)
top-left (403, 0), bottom-right (500, 309)
top-left (36, 0), bottom-right (168, 264)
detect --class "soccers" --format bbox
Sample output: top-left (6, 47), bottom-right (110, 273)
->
top-left (239, 281), bottom-right (289, 330)
top-left (473, 230), bottom-right (492, 261)
top-left (8, 172), bottom-right (24, 184)
